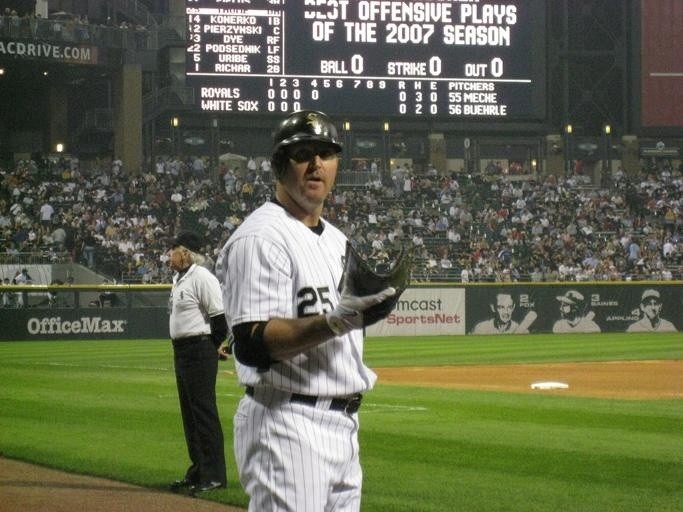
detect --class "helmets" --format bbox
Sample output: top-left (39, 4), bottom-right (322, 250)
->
top-left (269, 110), bottom-right (345, 159)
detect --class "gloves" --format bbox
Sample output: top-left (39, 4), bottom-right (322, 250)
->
top-left (325, 271), bottom-right (398, 338)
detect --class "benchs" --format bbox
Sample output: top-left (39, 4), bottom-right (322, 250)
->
top-left (0, 138), bottom-right (682, 290)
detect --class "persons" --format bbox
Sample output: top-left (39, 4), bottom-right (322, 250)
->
top-left (466, 291), bottom-right (530, 334)
top-left (551, 290), bottom-right (603, 333)
top-left (0, 147), bottom-right (683, 282)
top-left (214, 106), bottom-right (397, 512)
top-left (623, 288), bottom-right (679, 333)
top-left (2, 7), bottom-right (147, 30)
top-left (166, 232), bottom-right (230, 494)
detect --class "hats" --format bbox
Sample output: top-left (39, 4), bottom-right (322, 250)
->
top-left (642, 289), bottom-right (662, 303)
top-left (162, 231), bottom-right (206, 254)
top-left (556, 290), bottom-right (585, 307)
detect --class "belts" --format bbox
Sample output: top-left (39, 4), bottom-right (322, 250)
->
top-left (243, 383), bottom-right (364, 416)
top-left (173, 336), bottom-right (214, 345)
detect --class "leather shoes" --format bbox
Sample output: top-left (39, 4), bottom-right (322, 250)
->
top-left (191, 481), bottom-right (227, 492)
top-left (168, 479), bottom-right (197, 487)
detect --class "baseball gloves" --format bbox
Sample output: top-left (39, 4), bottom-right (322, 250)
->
top-left (324, 236), bottom-right (411, 337)
